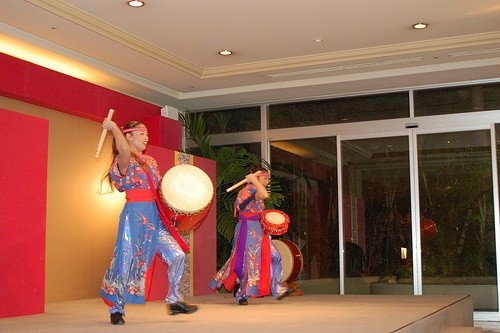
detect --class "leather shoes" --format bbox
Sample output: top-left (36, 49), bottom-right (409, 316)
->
top-left (169, 302), bottom-right (198, 315)
top-left (110, 312), bottom-right (125, 325)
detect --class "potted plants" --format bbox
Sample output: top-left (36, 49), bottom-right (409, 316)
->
top-left (367, 168), bottom-right (411, 295)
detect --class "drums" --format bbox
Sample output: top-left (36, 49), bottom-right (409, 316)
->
top-left (271, 239), bottom-right (304, 284)
top-left (419, 218), bottom-right (437, 244)
top-left (156, 164), bottom-right (215, 235)
top-left (261, 210), bottom-right (290, 235)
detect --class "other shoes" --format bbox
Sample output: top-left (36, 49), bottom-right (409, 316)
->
top-left (239, 298), bottom-right (248, 305)
top-left (277, 288), bottom-right (295, 301)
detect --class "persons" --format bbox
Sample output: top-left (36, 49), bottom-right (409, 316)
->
top-left (100, 119), bottom-right (199, 325)
top-left (208, 164), bottom-right (300, 306)
